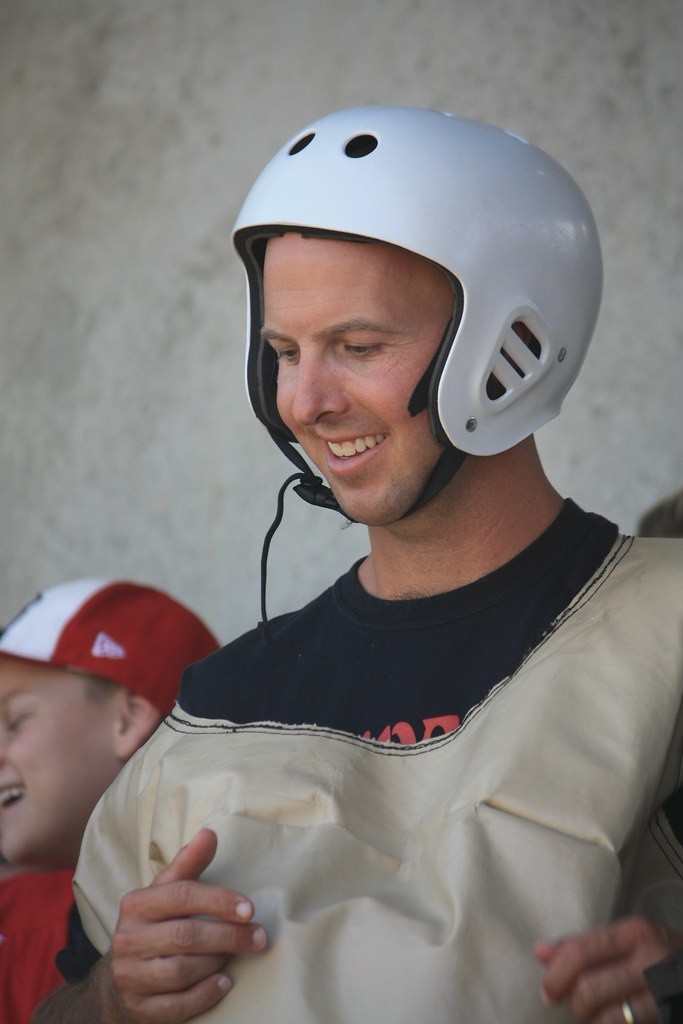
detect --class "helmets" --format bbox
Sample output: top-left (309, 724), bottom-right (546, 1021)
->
top-left (228, 100), bottom-right (606, 461)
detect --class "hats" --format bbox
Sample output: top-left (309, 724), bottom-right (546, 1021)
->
top-left (0, 577), bottom-right (223, 720)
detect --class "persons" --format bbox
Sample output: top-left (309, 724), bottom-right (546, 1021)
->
top-left (18, 107), bottom-right (683, 1024)
top-left (0, 580), bottom-right (230, 1024)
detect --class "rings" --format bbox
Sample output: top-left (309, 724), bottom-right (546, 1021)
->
top-left (622, 1000), bottom-right (634, 1024)
